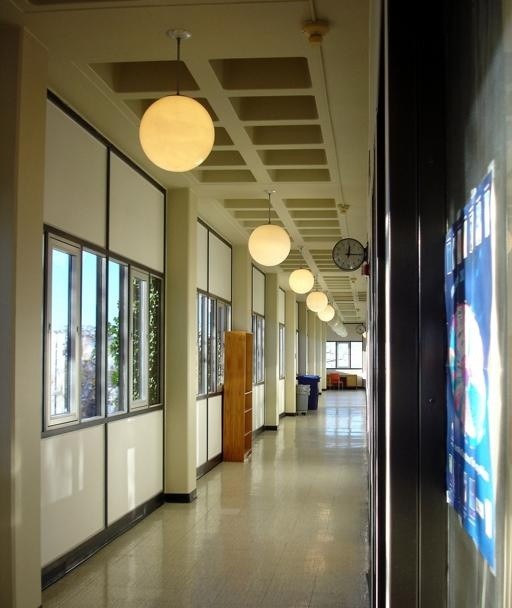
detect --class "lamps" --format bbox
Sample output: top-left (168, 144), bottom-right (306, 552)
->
top-left (318, 305), bottom-right (335, 322)
top-left (306, 275), bottom-right (328, 313)
top-left (248, 189), bottom-right (291, 267)
top-left (327, 316), bottom-right (348, 337)
top-left (139, 30), bottom-right (215, 172)
top-left (288, 246), bottom-right (314, 294)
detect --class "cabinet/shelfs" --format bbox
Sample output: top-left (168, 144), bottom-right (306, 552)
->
top-left (223, 331), bottom-right (253, 461)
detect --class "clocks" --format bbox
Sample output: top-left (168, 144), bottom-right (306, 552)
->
top-left (332, 238), bottom-right (368, 271)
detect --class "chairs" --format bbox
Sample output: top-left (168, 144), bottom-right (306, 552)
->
top-left (331, 374), bottom-right (343, 391)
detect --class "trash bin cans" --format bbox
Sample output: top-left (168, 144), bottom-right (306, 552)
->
top-left (297, 374), bottom-right (320, 414)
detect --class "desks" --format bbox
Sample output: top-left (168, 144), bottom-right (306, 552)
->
top-left (327, 375), bottom-right (357, 390)
